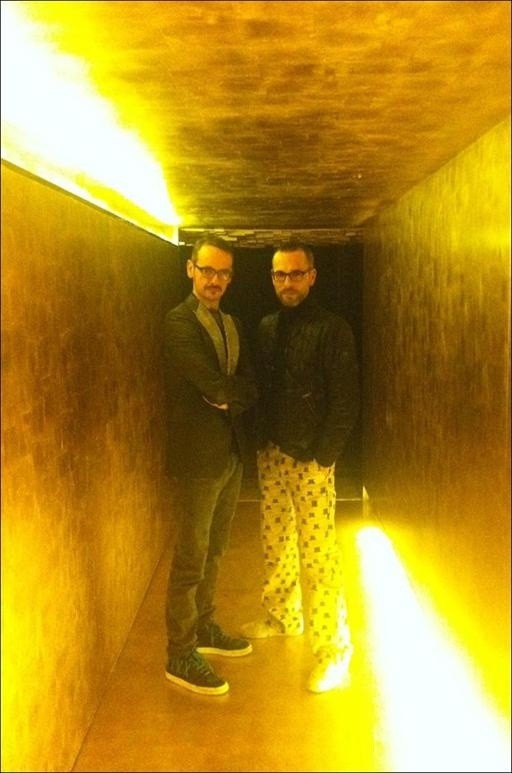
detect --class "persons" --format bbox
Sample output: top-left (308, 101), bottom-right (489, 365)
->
top-left (161, 235), bottom-right (265, 695)
top-left (238, 243), bottom-right (362, 694)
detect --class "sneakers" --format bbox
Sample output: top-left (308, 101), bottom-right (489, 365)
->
top-left (164, 657), bottom-right (230, 697)
top-left (302, 647), bottom-right (352, 694)
top-left (240, 616), bottom-right (304, 639)
top-left (194, 622), bottom-right (253, 657)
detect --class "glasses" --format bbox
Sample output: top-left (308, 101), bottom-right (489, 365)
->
top-left (193, 262), bottom-right (233, 281)
top-left (270, 268), bottom-right (315, 282)
